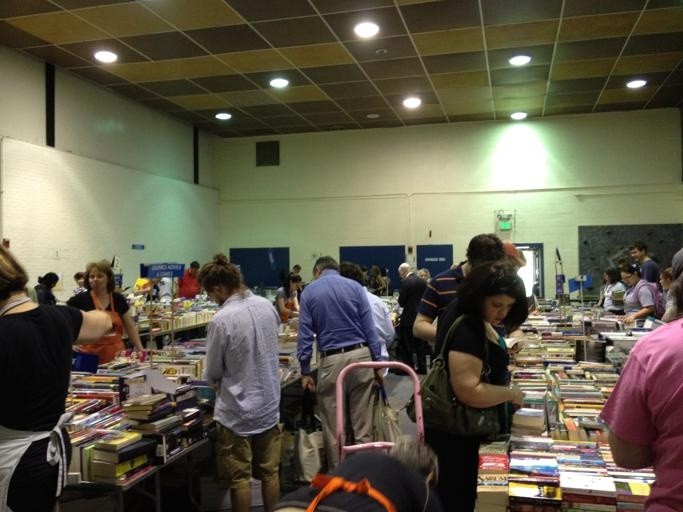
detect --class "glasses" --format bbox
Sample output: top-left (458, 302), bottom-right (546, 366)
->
top-left (622, 274), bottom-right (633, 282)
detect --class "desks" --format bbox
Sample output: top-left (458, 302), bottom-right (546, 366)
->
top-left (121, 300), bottom-right (209, 355)
top-left (66, 341), bottom-right (316, 512)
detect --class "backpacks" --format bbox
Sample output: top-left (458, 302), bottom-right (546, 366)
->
top-left (634, 282), bottom-right (667, 320)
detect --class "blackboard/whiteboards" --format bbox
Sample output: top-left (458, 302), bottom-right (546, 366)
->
top-left (578, 223), bottom-right (683, 301)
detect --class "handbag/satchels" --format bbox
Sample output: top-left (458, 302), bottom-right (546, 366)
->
top-left (370, 375), bottom-right (402, 454)
top-left (405, 313), bottom-right (501, 437)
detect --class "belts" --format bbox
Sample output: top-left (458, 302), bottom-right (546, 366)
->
top-left (320, 342), bottom-right (368, 359)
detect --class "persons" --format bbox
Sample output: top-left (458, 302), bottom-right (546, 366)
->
top-left (0, 243), bottom-right (114, 512)
top-left (197, 251), bottom-right (283, 512)
top-left (266, 234), bottom-right (528, 512)
top-left (72, 271), bottom-right (88, 295)
top-left (66, 259), bottom-right (144, 366)
top-left (132, 277), bottom-right (164, 350)
top-left (33, 271), bottom-right (59, 306)
top-left (178, 260), bottom-right (201, 299)
top-left (593, 238), bottom-right (683, 512)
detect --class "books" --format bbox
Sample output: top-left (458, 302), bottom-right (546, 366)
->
top-left (64, 296), bottom-right (219, 486)
top-left (471, 294), bottom-right (658, 512)
